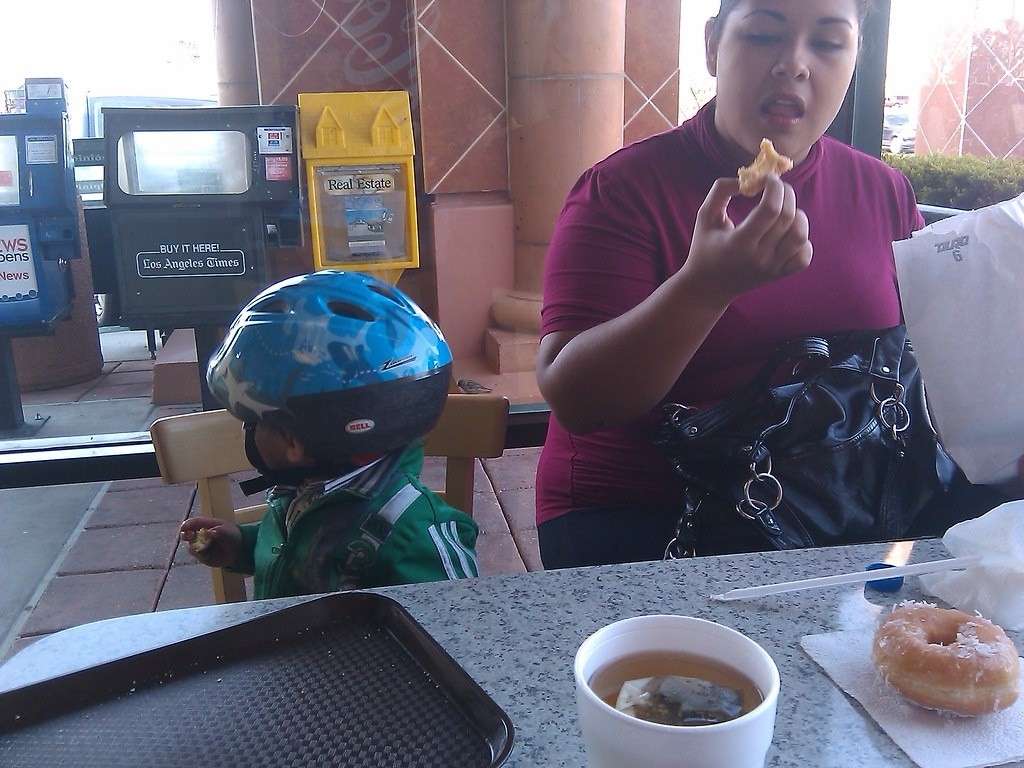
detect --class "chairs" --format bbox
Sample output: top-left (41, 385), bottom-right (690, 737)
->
top-left (146, 396), bottom-right (511, 606)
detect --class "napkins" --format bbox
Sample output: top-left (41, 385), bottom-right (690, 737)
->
top-left (799, 627), bottom-right (1024, 768)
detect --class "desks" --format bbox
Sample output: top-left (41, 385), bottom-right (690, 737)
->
top-left (0, 533), bottom-right (1024, 768)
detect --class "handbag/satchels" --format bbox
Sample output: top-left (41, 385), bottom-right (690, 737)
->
top-left (657, 323), bottom-right (980, 560)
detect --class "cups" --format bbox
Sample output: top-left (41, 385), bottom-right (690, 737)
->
top-left (574, 614), bottom-right (780, 768)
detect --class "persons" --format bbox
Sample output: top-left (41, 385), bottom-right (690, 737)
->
top-left (180, 267), bottom-right (478, 602)
top-left (532, 0), bottom-right (930, 574)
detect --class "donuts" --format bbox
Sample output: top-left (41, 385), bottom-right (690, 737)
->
top-left (872, 599), bottom-right (1022, 720)
top-left (736, 138), bottom-right (793, 197)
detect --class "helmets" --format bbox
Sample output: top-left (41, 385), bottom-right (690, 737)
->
top-left (208, 270), bottom-right (452, 453)
